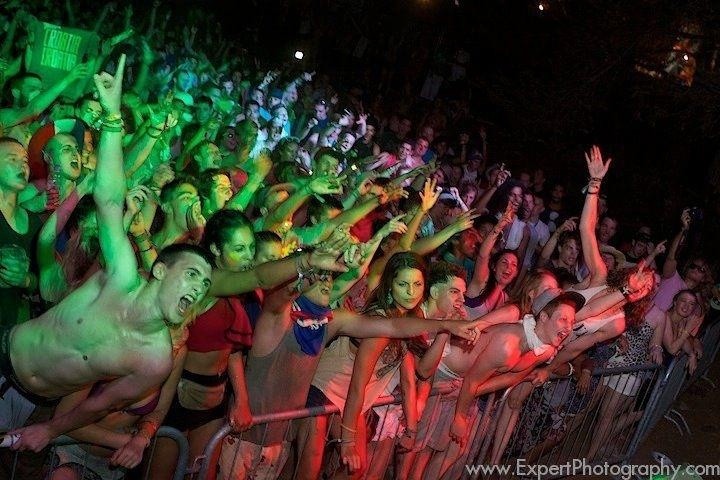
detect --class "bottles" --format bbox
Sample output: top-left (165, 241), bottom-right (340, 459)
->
top-left (48, 165), bottom-right (66, 204)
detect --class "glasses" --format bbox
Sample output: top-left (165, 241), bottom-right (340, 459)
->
top-left (688, 263), bottom-right (706, 274)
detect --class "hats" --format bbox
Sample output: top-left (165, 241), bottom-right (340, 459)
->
top-left (529, 287), bottom-right (587, 316)
top-left (27, 119), bottom-right (85, 175)
top-left (599, 244), bottom-right (627, 272)
